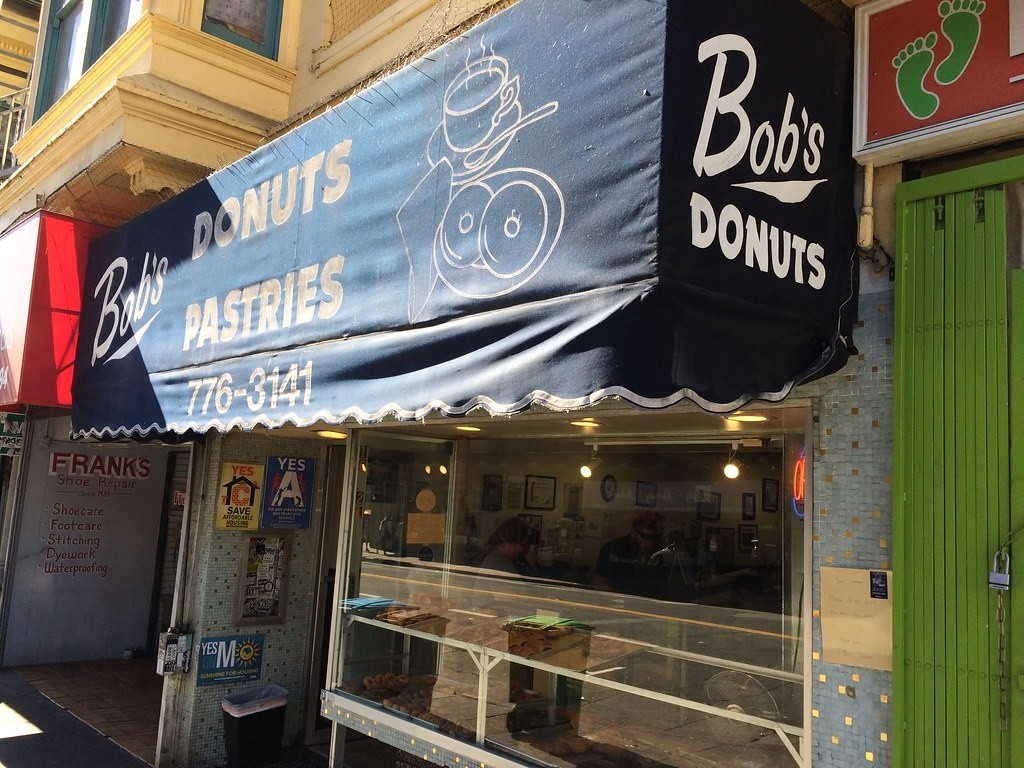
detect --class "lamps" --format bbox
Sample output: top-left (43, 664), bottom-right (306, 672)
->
top-left (578, 442), bottom-right (606, 477)
top-left (722, 437), bottom-right (752, 480)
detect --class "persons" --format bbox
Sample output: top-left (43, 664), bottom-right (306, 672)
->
top-left (471, 518), bottom-right (540, 612)
top-left (592, 512), bottom-right (668, 599)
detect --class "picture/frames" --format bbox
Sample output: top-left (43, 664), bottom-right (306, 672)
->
top-left (519, 514), bottom-right (544, 538)
top-left (706, 527), bottom-right (736, 564)
top-left (482, 475), bottom-right (504, 511)
top-left (636, 480), bottom-right (659, 508)
top-left (600, 475), bottom-right (619, 502)
top-left (563, 482), bottom-right (583, 514)
top-left (508, 483), bottom-right (523, 509)
top-left (696, 490), bottom-right (722, 521)
top-left (738, 523), bottom-right (758, 552)
top-left (584, 511), bottom-right (608, 538)
top-left (741, 493), bottom-right (757, 518)
top-left (524, 475), bottom-right (558, 510)
top-left (761, 479), bottom-right (779, 511)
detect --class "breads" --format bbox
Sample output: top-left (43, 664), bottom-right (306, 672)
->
top-left (362, 591), bottom-right (624, 758)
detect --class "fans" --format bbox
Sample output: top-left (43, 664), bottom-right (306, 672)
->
top-left (701, 670), bottom-right (781, 744)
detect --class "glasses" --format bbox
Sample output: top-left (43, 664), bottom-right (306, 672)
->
top-left (632, 526), bottom-right (655, 540)
top-left (519, 542), bottom-right (530, 551)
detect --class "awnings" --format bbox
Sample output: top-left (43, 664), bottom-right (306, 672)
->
top-left (69, 0), bottom-right (861, 438)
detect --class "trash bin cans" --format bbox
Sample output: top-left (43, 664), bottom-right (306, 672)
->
top-left (339, 598), bottom-right (421, 687)
top-left (221, 684), bottom-right (290, 768)
top-left (499, 616), bottom-right (595, 740)
top-left (372, 608), bottom-right (451, 711)
